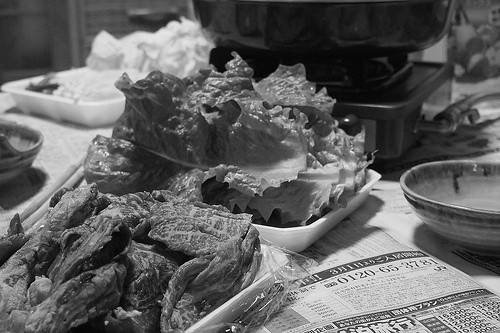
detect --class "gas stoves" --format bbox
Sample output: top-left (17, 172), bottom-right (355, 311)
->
top-left (208, 48), bottom-right (455, 159)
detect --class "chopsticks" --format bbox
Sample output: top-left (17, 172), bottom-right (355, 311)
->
top-left (17, 155), bottom-right (85, 231)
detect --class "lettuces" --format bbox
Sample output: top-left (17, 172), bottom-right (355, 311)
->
top-left (0, 51), bottom-right (380, 333)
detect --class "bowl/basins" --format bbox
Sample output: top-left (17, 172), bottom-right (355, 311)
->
top-left (0, 118), bottom-right (44, 185)
top-left (400, 160), bottom-right (500, 256)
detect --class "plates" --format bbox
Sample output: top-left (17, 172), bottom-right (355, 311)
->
top-left (0, 67), bottom-right (154, 127)
top-left (184, 243), bottom-right (289, 333)
top-left (250, 168), bottom-right (382, 253)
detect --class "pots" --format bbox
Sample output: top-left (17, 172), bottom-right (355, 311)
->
top-left (191, 0), bottom-right (454, 57)
